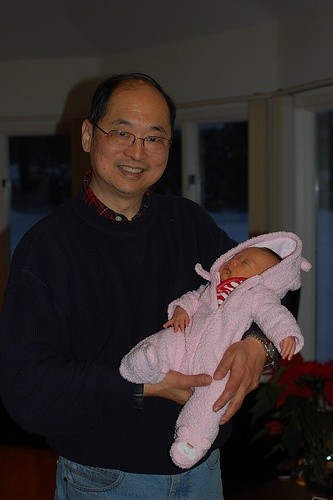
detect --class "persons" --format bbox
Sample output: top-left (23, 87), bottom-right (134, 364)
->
top-left (0, 71), bottom-right (302, 500)
top-left (119, 247), bottom-right (305, 470)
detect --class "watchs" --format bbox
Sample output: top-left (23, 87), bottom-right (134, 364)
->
top-left (241, 329), bottom-right (277, 367)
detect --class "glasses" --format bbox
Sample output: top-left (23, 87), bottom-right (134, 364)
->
top-left (89, 121), bottom-right (174, 155)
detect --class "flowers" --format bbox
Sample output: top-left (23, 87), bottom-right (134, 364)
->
top-left (248, 354), bottom-right (333, 491)
